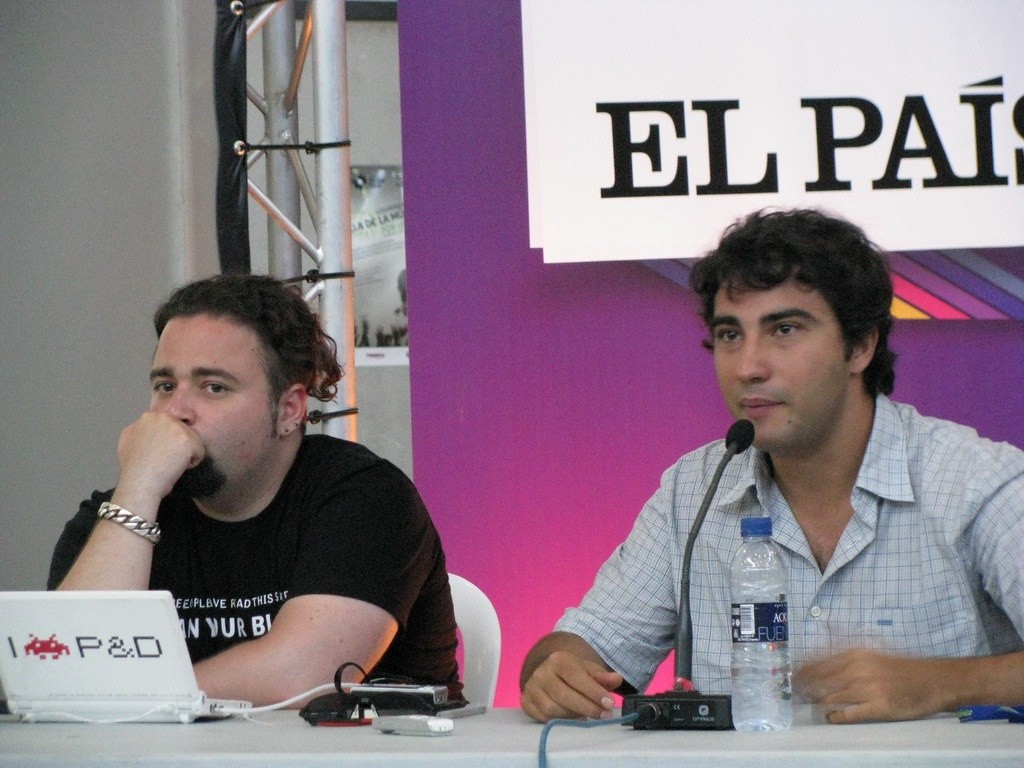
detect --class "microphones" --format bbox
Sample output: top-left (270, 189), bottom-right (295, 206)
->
top-left (620, 418), bottom-right (756, 731)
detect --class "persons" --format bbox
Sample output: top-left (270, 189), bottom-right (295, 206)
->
top-left (44, 272), bottom-right (471, 712)
top-left (518, 207), bottom-right (1024, 725)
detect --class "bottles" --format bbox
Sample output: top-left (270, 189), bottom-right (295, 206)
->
top-left (727, 517), bottom-right (793, 731)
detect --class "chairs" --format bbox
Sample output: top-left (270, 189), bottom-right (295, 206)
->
top-left (448, 571), bottom-right (501, 708)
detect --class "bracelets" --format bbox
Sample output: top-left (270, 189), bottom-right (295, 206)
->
top-left (98, 502), bottom-right (161, 544)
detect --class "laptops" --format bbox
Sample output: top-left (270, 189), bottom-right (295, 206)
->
top-left (0, 590), bottom-right (252, 725)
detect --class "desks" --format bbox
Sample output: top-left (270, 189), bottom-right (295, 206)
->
top-left (0, 712), bottom-right (1024, 768)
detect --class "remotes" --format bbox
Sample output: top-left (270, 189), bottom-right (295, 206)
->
top-left (352, 684), bottom-right (448, 706)
top-left (372, 715), bottom-right (453, 738)
